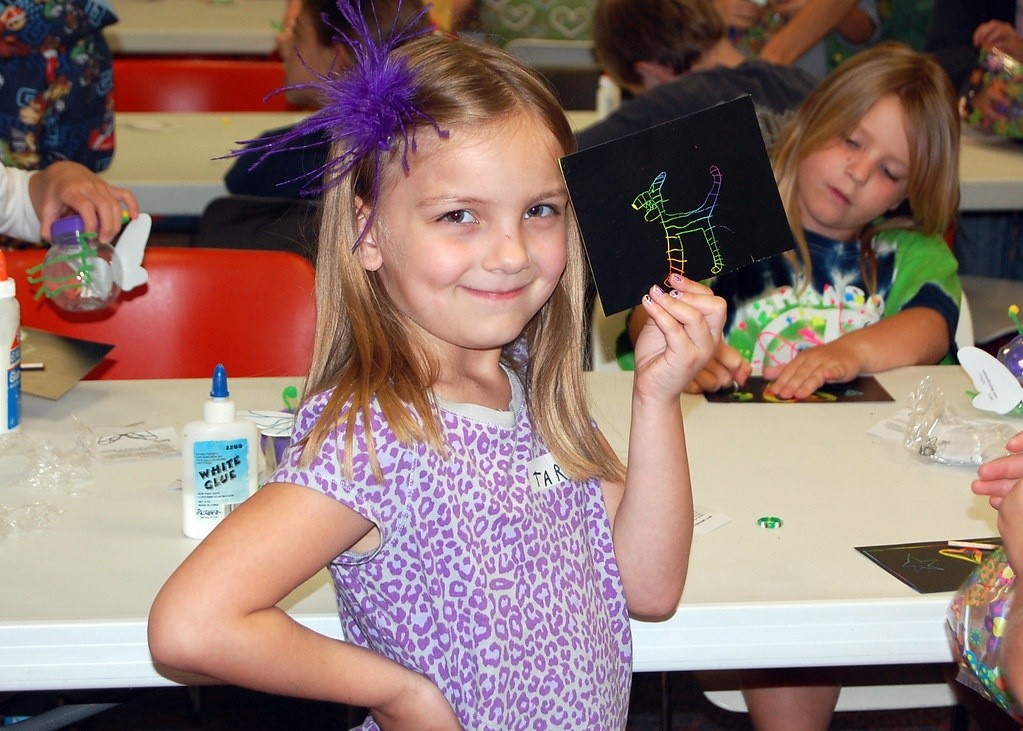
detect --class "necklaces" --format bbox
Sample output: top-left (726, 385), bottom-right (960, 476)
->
top-left (571, 0), bottom-right (884, 152)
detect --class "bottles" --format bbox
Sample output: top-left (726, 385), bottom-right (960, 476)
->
top-left (0, 249), bottom-right (20, 434)
top-left (596, 68), bottom-right (622, 123)
top-left (42, 214), bottom-right (124, 312)
top-left (179, 363), bottom-right (260, 540)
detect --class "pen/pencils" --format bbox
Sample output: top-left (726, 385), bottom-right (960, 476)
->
top-left (718, 331), bottom-right (739, 390)
top-left (20, 362), bottom-right (42, 367)
top-left (947, 539), bottom-right (1000, 550)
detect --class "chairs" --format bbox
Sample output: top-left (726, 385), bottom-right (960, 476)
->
top-left (1, 230), bottom-right (321, 384)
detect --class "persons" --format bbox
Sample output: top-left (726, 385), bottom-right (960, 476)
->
top-left (972, 432), bottom-right (1022, 707)
top-left (625, 49), bottom-right (961, 731)
top-left (0, 162), bottom-right (140, 246)
top-left (0, 0), bottom-right (120, 174)
top-left (147, 34), bottom-right (728, 731)
top-left (952, 0), bottom-right (1023, 276)
top-left (223, 0), bottom-right (434, 204)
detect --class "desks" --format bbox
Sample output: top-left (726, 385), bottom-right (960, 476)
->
top-left (101, 113), bottom-right (1022, 220)
top-left (0, 361), bottom-right (1023, 730)
